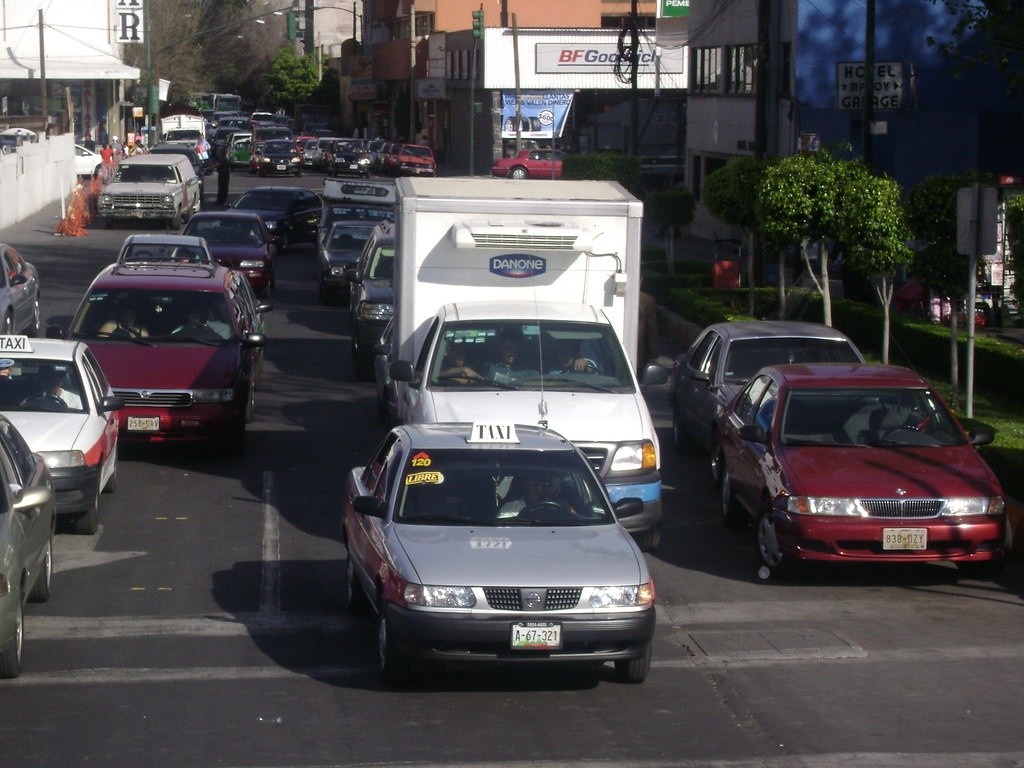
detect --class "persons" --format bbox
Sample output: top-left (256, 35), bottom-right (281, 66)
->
top-left (440, 328), bottom-right (535, 384)
top-left (100, 131), bottom-right (146, 163)
top-left (178, 248), bottom-right (191, 262)
top-left (547, 342), bottom-right (597, 374)
top-left (20, 365), bottom-right (83, 409)
top-left (171, 301), bottom-right (230, 339)
top-left (215, 157), bottom-right (234, 206)
top-left (195, 134), bottom-right (211, 175)
top-left (495, 471), bottom-right (575, 518)
top-left (97, 306), bottom-right (149, 338)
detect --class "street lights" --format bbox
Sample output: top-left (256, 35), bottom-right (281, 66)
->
top-left (964, 169), bottom-right (1024, 417)
top-left (309, 5), bottom-right (363, 47)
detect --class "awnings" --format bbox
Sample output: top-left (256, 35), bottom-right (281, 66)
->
top-left (0, 62), bottom-right (142, 80)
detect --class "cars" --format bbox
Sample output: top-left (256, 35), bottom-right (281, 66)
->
top-left (0, 240), bottom-right (125, 679)
top-left (670, 321), bottom-right (868, 462)
top-left (75, 109), bottom-right (438, 202)
top-left (224, 186), bottom-right (324, 254)
top-left (712, 366), bottom-right (1008, 580)
top-left (64, 210), bottom-right (274, 464)
top-left (344, 425), bottom-right (657, 688)
top-left (319, 219), bottom-right (382, 306)
top-left (372, 315), bottom-right (394, 423)
top-left (490, 148), bottom-right (568, 181)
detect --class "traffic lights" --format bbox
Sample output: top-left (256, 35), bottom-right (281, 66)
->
top-left (295, 12), bottom-right (306, 38)
top-left (472, 10), bottom-right (481, 39)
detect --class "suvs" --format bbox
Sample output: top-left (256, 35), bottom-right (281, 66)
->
top-left (347, 221), bottom-right (396, 377)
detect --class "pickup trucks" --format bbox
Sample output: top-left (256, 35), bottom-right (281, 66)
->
top-left (386, 176), bottom-right (665, 539)
top-left (98, 154), bottom-right (202, 229)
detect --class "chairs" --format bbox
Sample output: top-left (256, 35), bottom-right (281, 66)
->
top-left (864, 405), bottom-right (910, 444)
top-left (335, 234), bottom-right (353, 248)
top-left (418, 462), bottom-right (496, 523)
top-left (40, 365), bottom-right (71, 389)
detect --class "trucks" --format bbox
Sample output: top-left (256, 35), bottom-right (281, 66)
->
top-left (322, 179), bottom-right (398, 233)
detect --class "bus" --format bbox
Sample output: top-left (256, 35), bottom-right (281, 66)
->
top-left (294, 104), bottom-right (327, 129)
top-left (186, 91), bottom-right (241, 116)
